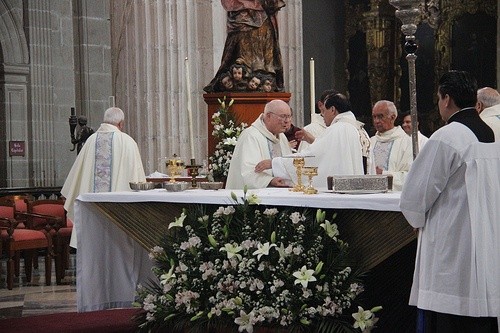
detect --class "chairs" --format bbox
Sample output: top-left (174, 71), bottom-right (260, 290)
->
top-left (0, 200), bottom-right (59, 290)
top-left (24, 197), bottom-right (73, 286)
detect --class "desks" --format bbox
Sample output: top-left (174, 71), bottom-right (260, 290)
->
top-left (74, 189), bottom-right (420, 333)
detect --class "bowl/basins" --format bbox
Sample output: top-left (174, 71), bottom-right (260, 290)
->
top-left (165, 184), bottom-right (182, 191)
top-left (200, 182), bottom-right (224, 189)
top-left (129, 182), bottom-right (152, 190)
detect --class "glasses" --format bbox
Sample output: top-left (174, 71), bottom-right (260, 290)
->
top-left (270, 112), bottom-right (292, 120)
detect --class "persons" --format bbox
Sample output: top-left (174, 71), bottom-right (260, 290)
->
top-left (203, 0), bottom-right (287, 94)
top-left (71, 115), bottom-right (93, 156)
top-left (284, 89), bottom-right (376, 187)
top-left (399, 70), bottom-right (500, 333)
top-left (60, 107), bottom-right (152, 313)
top-left (224, 100), bottom-right (295, 190)
top-left (370, 100), bottom-right (413, 191)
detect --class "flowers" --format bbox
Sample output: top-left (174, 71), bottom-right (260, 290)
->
top-left (130, 184), bottom-right (383, 333)
top-left (201, 96), bottom-right (248, 189)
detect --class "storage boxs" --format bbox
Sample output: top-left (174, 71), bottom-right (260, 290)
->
top-left (327, 175), bottom-right (394, 194)
